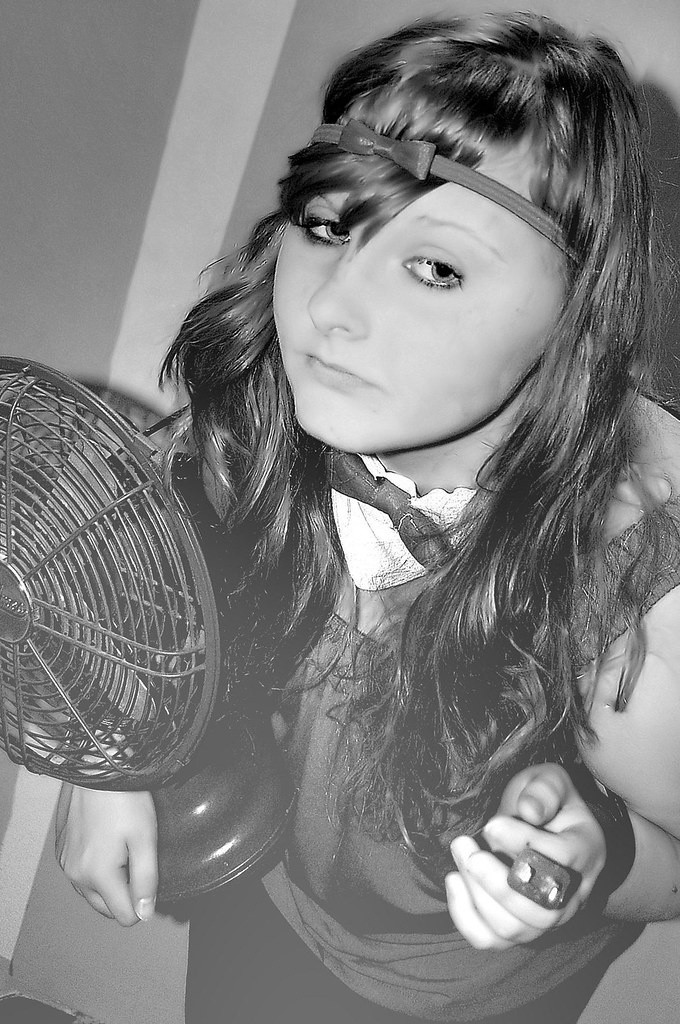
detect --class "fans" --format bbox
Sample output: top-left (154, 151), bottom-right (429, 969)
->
top-left (0, 355), bottom-right (292, 907)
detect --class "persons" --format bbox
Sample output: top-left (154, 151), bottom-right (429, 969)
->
top-left (54, 12), bottom-right (680, 1024)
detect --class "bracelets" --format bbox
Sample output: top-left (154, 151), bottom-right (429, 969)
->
top-left (583, 781), bottom-right (635, 915)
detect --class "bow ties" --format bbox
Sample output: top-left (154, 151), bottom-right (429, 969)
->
top-left (327, 450), bottom-right (453, 570)
